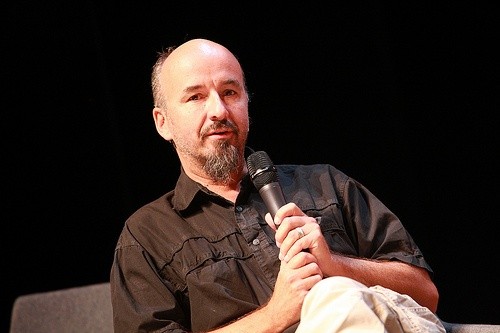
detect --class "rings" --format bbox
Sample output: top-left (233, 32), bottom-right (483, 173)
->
top-left (296, 227), bottom-right (304, 238)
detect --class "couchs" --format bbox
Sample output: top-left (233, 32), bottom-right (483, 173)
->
top-left (10, 283), bottom-right (500, 333)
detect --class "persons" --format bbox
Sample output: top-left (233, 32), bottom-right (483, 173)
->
top-left (110, 38), bottom-right (447, 333)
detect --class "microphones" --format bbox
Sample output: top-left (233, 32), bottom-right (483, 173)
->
top-left (247, 151), bottom-right (317, 291)
